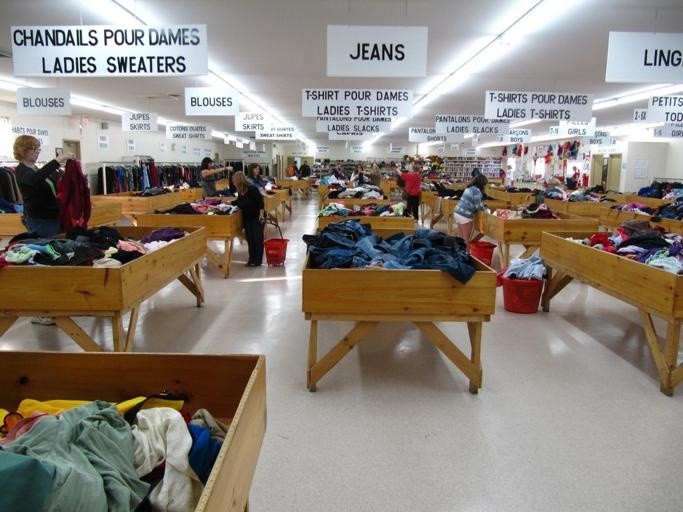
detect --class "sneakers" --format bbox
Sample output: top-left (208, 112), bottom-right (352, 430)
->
top-left (29, 315), bottom-right (57, 326)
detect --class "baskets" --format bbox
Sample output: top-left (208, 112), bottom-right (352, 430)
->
top-left (468, 240), bottom-right (497, 266)
top-left (501, 272), bottom-right (543, 314)
top-left (260, 218), bottom-right (288, 266)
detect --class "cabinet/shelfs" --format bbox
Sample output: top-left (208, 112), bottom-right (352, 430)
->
top-left (440, 156), bottom-right (503, 179)
top-left (1, 224), bottom-right (207, 353)
top-left (541, 230), bottom-right (682, 397)
top-left (318, 185), bottom-right (414, 238)
top-left (421, 188), bottom-right (683, 271)
top-left (1, 351), bottom-right (267, 512)
top-left (303, 229), bottom-right (497, 394)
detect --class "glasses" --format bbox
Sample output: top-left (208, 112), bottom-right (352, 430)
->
top-left (27, 148), bottom-right (41, 154)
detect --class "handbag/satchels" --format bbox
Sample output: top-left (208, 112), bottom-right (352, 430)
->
top-left (401, 190), bottom-right (410, 201)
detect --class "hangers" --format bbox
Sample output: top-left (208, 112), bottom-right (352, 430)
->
top-left (102, 161), bottom-right (270, 169)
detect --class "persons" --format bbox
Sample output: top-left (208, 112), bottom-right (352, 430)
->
top-left (248, 163), bottom-right (264, 182)
top-left (200, 157), bottom-right (234, 195)
top-left (285, 160), bottom-right (310, 177)
top-left (453, 174), bottom-right (488, 255)
top-left (13, 132), bottom-right (77, 326)
top-left (499, 168), bottom-right (506, 183)
top-left (222, 170), bottom-right (267, 267)
top-left (350, 162), bottom-right (422, 220)
top-left (572, 166), bottom-right (580, 181)
